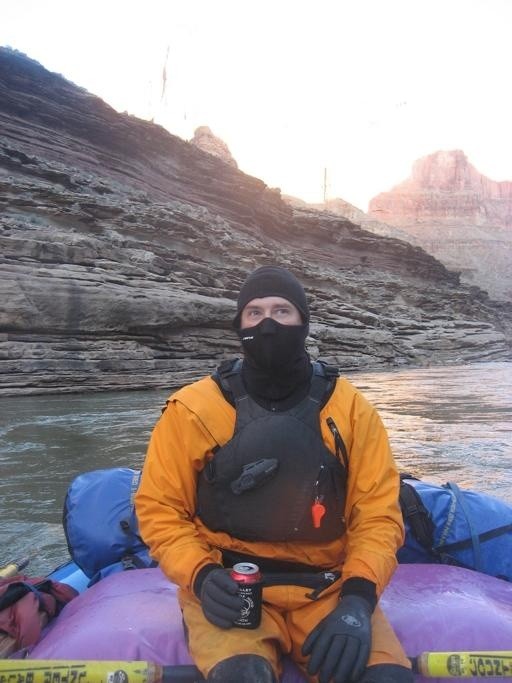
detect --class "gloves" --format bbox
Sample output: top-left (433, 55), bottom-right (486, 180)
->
top-left (301, 574), bottom-right (378, 683)
top-left (194, 560), bottom-right (245, 629)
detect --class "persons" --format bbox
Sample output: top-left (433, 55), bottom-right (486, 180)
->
top-left (133, 265), bottom-right (419, 682)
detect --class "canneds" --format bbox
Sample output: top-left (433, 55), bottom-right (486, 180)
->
top-left (230, 562), bottom-right (263, 629)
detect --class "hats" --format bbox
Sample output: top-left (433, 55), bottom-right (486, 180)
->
top-left (232, 265), bottom-right (313, 413)
